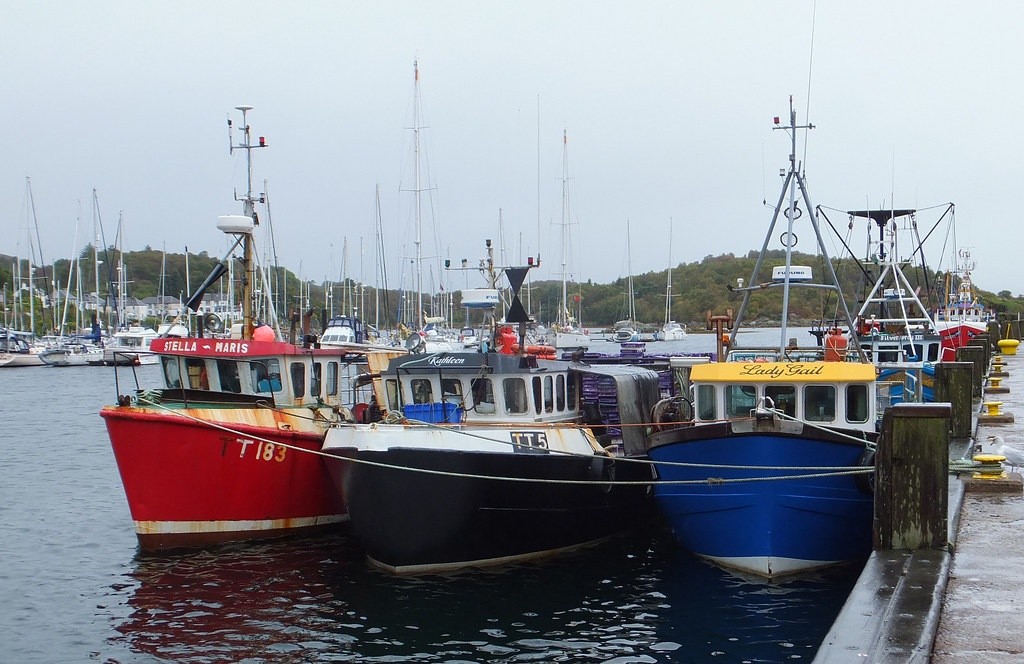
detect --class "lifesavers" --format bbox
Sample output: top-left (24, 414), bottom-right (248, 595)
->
top-left (729, 358), bottom-right (770, 363)
top-left (510, 343), bottom-right (557, 355)
top-left (514, 353), bottom-right (558, 361)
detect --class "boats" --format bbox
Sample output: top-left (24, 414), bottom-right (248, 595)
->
top-left (807, 184), bottom-right (990, 409)
top-left (645, 5), bottom-right (882, 576)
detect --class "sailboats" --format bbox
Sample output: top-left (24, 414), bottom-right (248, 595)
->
top-left (0, 62), bottom-right (688, 576)
